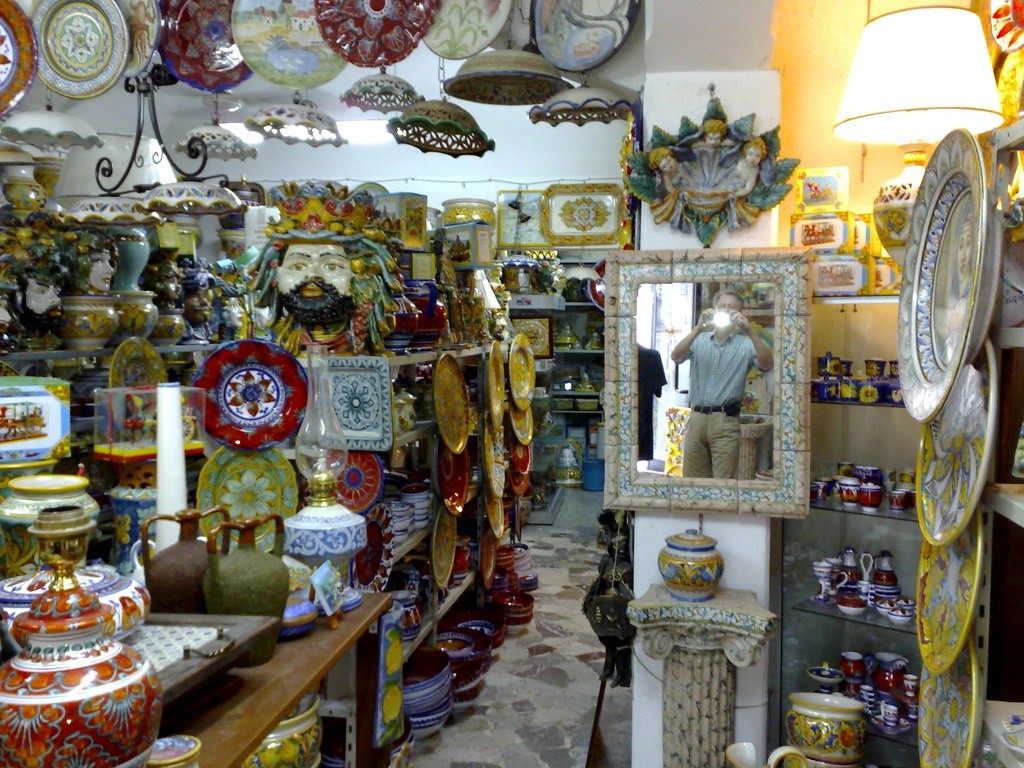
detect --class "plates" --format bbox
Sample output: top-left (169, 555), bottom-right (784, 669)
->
top-left (898, 127), bottom-right (1003, 768)
top-left (196, 444), bottom-right (298, 554)
top-left (188, 338), bottom-right (310, 452)
top-left (0, 0), bottom-right (638, 119)
top-left (300, 451), bottom-right (385, 517)
top-left (433, 333), bottom-right (536, 592)
top-left (325, 357), bottom-right (392, 452)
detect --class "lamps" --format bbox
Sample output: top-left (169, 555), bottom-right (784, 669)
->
top-left (51, 131), bottom-right (177, 292)
top-left (831, 4), bottom-right (1006, 295)
top-left (283, 344), bottom-right (368, 612)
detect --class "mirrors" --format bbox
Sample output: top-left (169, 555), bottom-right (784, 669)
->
top-left (602, 244), bottom-right (814, 520)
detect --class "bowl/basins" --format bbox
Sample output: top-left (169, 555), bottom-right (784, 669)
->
top-left (391, 487), bottom-right (540, 739)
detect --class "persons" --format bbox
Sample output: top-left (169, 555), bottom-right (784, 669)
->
top-left (9, 266), bottom-right (64, 338)
top-left (0, 290), bottom-right (21, 353)
top-left (214, 275), bottom-right (245, 344)
top-left (670, 289), bottom-right (774, 480)
top-left (141, 252), bottom-right (182, 311)
top-left (68, 232), bottom-right (118, 298)
top-left (179, 255), bottom-right (218, 345)
top-left (244, 178), bottom-right (401, 360)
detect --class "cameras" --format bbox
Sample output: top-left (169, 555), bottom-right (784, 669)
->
top-left (710, 312), bottom-right (735, 324)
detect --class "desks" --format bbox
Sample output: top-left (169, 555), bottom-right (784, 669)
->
top-left (159, 592), bottom-right (394, 768)
top-left (626, 584), bottom-right (779, 768)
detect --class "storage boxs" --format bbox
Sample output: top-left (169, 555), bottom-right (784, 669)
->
top-left (443, 218), bottom-right (494, 270)
top-left (374, 192), bottom-right (428, 252)
top-left (93, 387), bottom-right (206, 464)
top-left (0, 375), bottom-right (72, 465)
top-left (789, 166), bottom-right (904, 296)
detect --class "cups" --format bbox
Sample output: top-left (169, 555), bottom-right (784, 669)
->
top-left (806, 351), bottom-right (922, 735)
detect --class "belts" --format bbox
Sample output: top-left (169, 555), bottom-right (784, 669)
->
top-left (691, 401), bottom-right (741, 413)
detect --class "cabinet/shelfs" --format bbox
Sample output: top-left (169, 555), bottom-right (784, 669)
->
top-left (776, 292), bottom-right (920, 768)
top-left (295, 343), bottom-right (510, 768)
top-left (976, 112), bottom-right (1024, 768)
top-left (550, 257), bottom-right (606, 415)
top-left (508, 294), bottom-right (567, 526)
